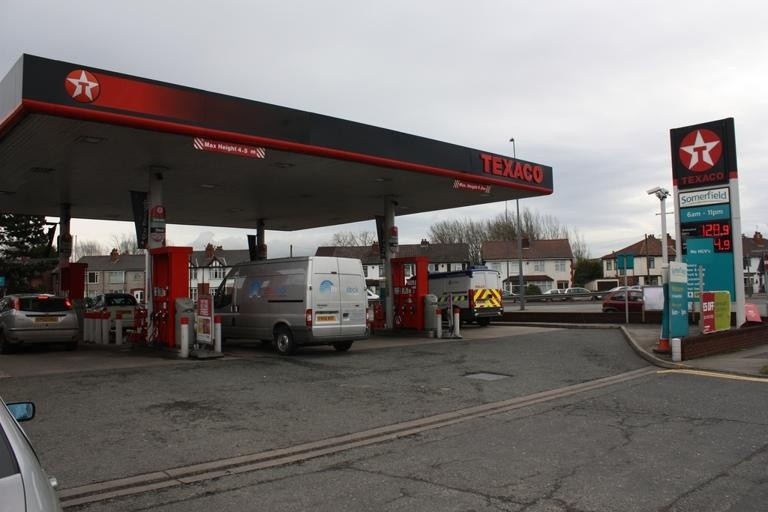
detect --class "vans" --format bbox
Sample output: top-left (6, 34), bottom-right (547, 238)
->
top-left (212, 256), bottom-right (371, 357)
top-left (405, 268), bottom-right (504, 328)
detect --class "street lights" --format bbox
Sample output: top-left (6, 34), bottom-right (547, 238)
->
top-left (509, 137), bottom-right (527, 311)
top-left (646, 185), bottom-right (671, 283)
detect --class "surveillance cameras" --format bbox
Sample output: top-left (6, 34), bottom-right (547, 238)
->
top-left (646, 186), bottom-right (670, 200)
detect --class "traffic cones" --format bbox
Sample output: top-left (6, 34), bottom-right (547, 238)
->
top-left (653, 321), bottom-right (672, 354)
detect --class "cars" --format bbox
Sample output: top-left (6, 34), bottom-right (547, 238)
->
top-left (92, 293), bottom-right (141, 330)
top-left (542, 284), bottom-right (650, 312)
top-left (0, 394), bottom-right (63, 511)
top-left (0, 293), bottom-right (80, 351)
top-left (367, 288), bottom-right (380, 300)
